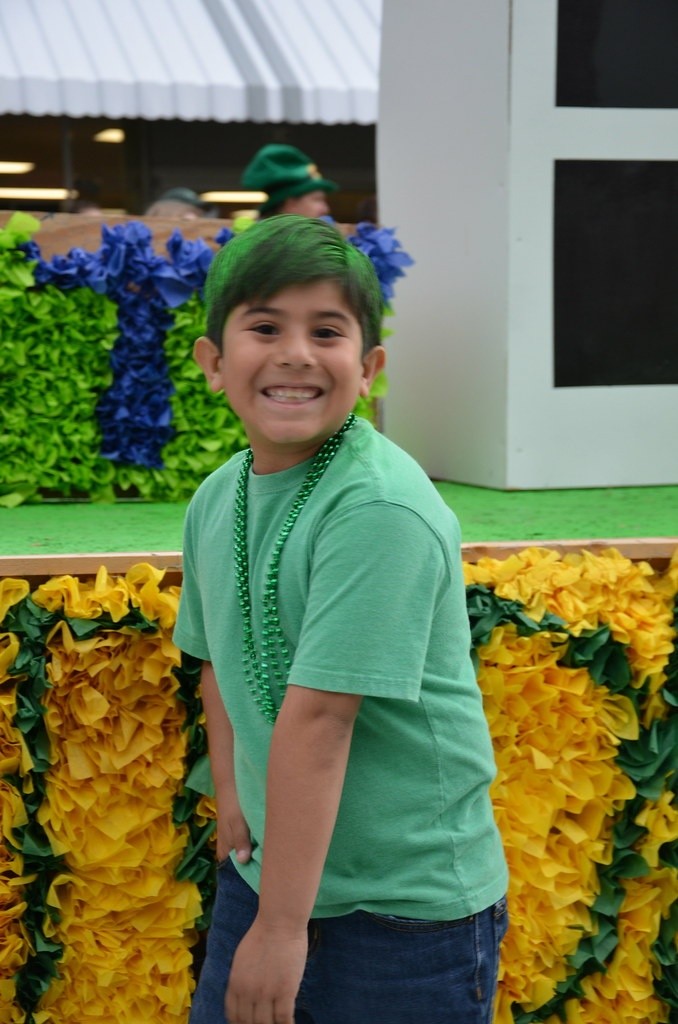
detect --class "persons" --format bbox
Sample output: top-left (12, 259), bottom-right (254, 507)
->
top-left (242, 144), bottom-right (333, 217)
top-left (172, 214), bottom-right (507, 1024)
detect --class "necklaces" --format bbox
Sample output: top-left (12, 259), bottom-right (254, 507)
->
top-left (232, 415), bottom-right (355, 725)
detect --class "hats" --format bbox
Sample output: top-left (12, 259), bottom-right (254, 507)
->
top-left (240, 143), bottom-right (341, 212)
